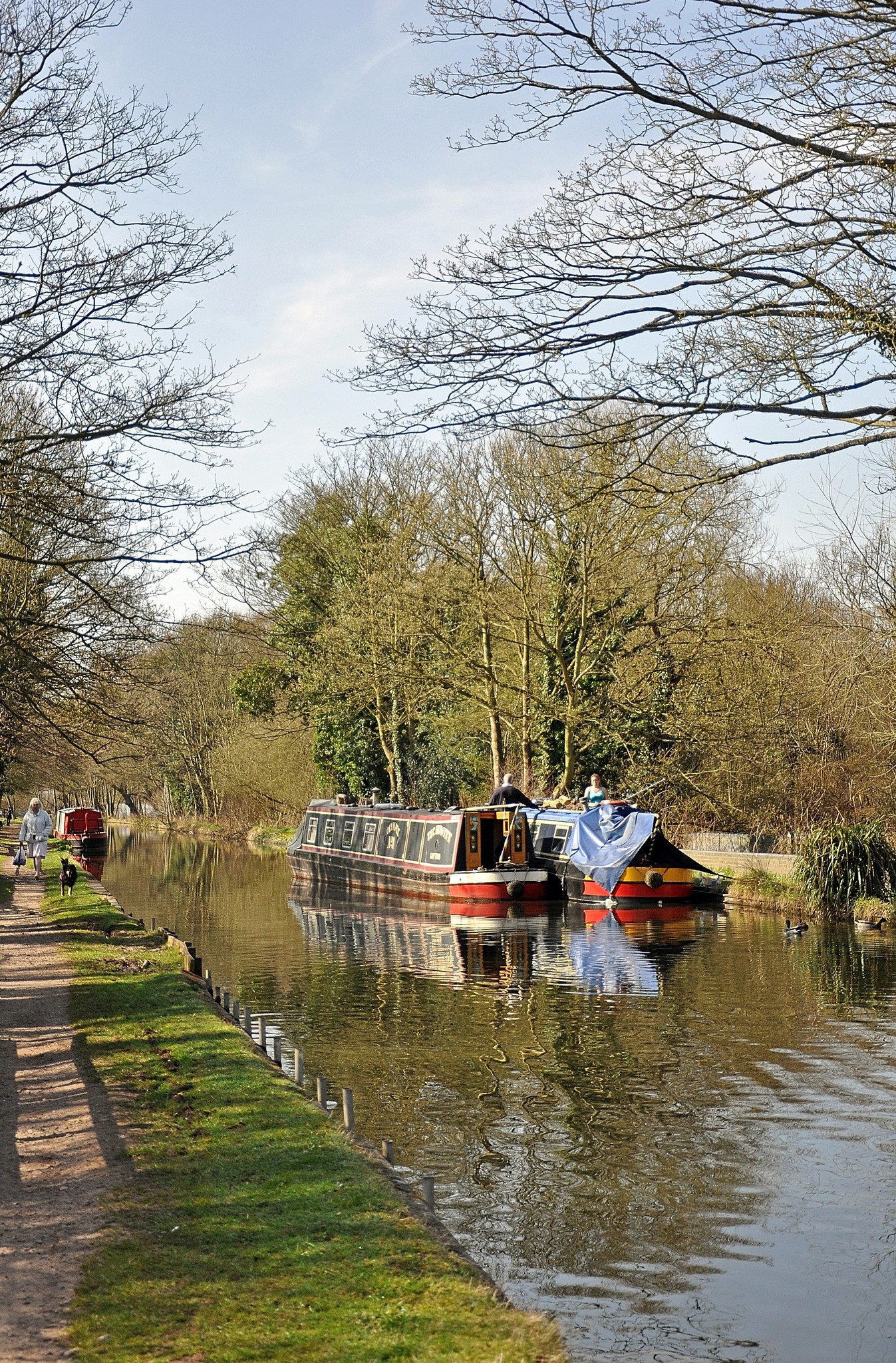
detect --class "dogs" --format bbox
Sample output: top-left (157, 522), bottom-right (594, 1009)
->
top-left (58, 856), bottom-right (77, 897)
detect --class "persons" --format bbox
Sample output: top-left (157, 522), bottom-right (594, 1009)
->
top-left (6, 807), bottom-right (12, 826)
top-left (584, 774), bottom-right (608, 804)
top-left (488, 773), bottom-right (544, 812)
top-left (18, 798), bottom-right (52, 880)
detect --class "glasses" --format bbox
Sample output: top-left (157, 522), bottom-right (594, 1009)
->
top-left (33, 804), bottom-right (38, 806)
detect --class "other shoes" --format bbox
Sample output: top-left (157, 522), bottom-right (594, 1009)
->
top-left (35, 874), bottom-right (39, 881)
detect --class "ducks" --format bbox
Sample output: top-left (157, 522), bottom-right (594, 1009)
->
top-left (783, 920), bottom-right (803, 934)
top-left (796, 919), bottom-right (809, 931)
top-left (854, 918), bottom-right (887, 931)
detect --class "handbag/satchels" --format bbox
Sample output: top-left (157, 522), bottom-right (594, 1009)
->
top-left (13, 843), bottom-right (26, 865)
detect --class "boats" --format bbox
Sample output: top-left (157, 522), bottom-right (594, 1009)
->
top-left (517, 806), bottom-right (695, 903)
top-left (284, 787), bottom-right (549, 903)
top-left (52, 806), bottom-right (107, 847)
